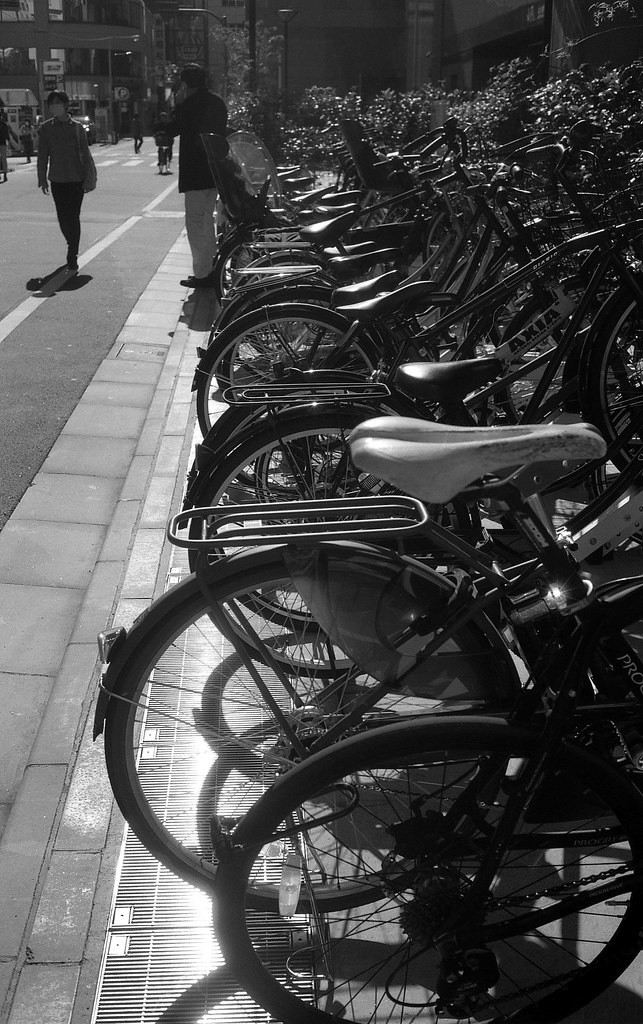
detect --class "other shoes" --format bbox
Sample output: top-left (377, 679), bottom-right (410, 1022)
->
top-left (180, 276), bottom-right (216, 289)
top-left (69, 256), bottom-right (78, 275)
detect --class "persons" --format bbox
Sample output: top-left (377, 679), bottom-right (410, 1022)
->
top-left (37, 90), bottom-right (98, 272)
top-left (154, 113), bottom-right (174, 167)
top-left (0, 112), bottom-right (16, 183)
top-left (167, 63), bottom-right (230, 289)
top-left (131, 113), bottom-right (143, 155)
top-left (20, 119), bottom-right (34, 163)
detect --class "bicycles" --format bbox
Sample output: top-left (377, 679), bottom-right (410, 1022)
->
top-left (87, 112), bottom-right (641, 1024)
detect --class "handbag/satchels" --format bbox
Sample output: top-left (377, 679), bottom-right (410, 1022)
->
top-left (81, 155), bottom-right (97, 192)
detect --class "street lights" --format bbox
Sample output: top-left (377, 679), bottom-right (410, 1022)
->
top-left (276, 8), bottom-right (299, 100)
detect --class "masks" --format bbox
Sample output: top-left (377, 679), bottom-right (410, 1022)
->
top-left (48, 103), bottom-right (65, 116)
